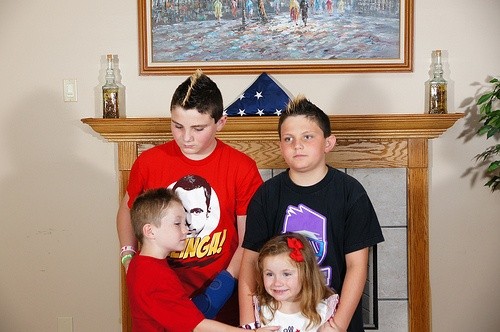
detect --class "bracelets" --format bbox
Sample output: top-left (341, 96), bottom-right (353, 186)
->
top-left (120, 245), bottom-right (138, 254)
top-left (121, 253), bottom-right (134, 265)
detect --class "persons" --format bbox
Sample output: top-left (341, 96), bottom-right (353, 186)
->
top-left (116, 70), bottom-right (266, 325)
top-left (258, 231), bottom-right (339, 332)
top-left (238, 96), bottom-right (384, 332)
top-left (170, 175), bottom-right (212, 240)
top-left (128, 187), bottom-right (281, 332)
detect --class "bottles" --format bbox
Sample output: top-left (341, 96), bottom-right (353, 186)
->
top-left (102, 55), bottom-right (120, 119)
top-left (428, 49), bottom-right (448, 114)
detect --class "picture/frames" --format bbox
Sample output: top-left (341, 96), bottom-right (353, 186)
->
top-left (138, 0), bottom-right (416, 74)
